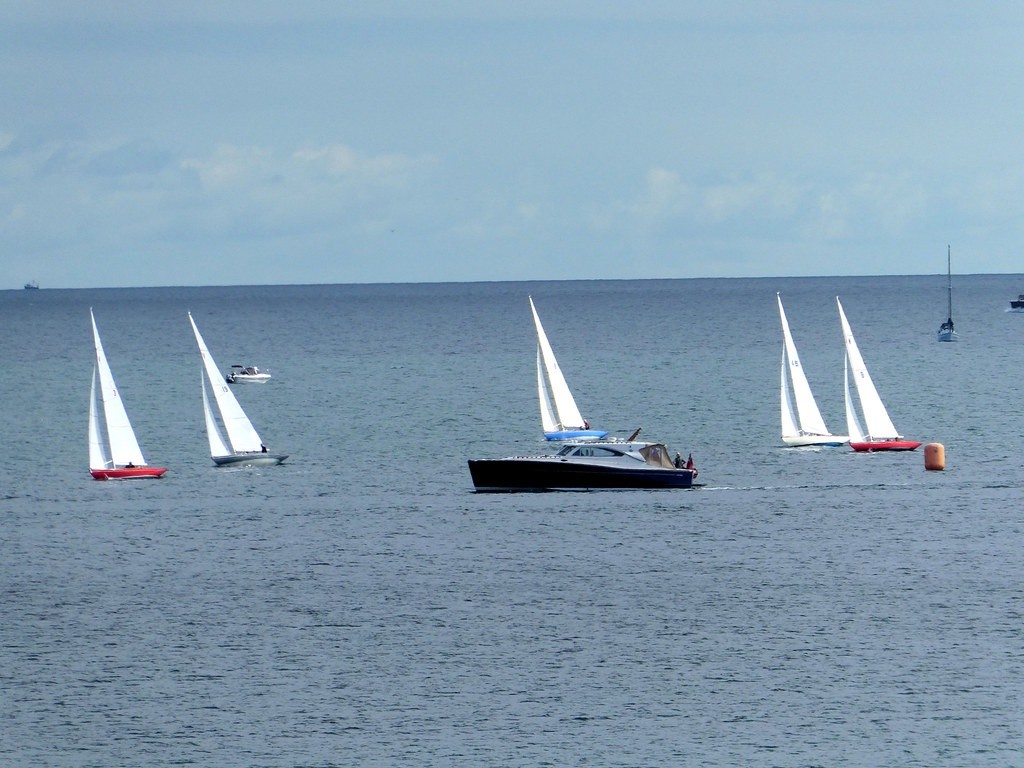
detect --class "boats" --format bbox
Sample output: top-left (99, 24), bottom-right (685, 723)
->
top-left (224, 361), bottom-right (276, 386)
top-left (1009, 293), bottom-right (1021, 310)
top-left (467, 422), bottom-right (699, 494)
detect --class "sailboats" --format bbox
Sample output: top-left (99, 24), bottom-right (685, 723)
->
top-left (188, 310), bottom-right (292, 467)
top-left (937, 242), bottom-right (958, 344)
top-left (87, 305), bottom-right (169, 479)
top-left (528, 295), bottom-right (610, 444)
top-left (775, 289), bottom-right (851, 448)
top-left (836, 295), bottom-right (925, 452)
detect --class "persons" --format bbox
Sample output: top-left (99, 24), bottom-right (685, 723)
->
top-left (585, 420), bottom-right (590, 430)
top-left (673, 454), bottom-right (686, 468)
top-left (126, 462), bottom-right (135, 468)
top-left (260, 444), bottom-right (267, 453)
